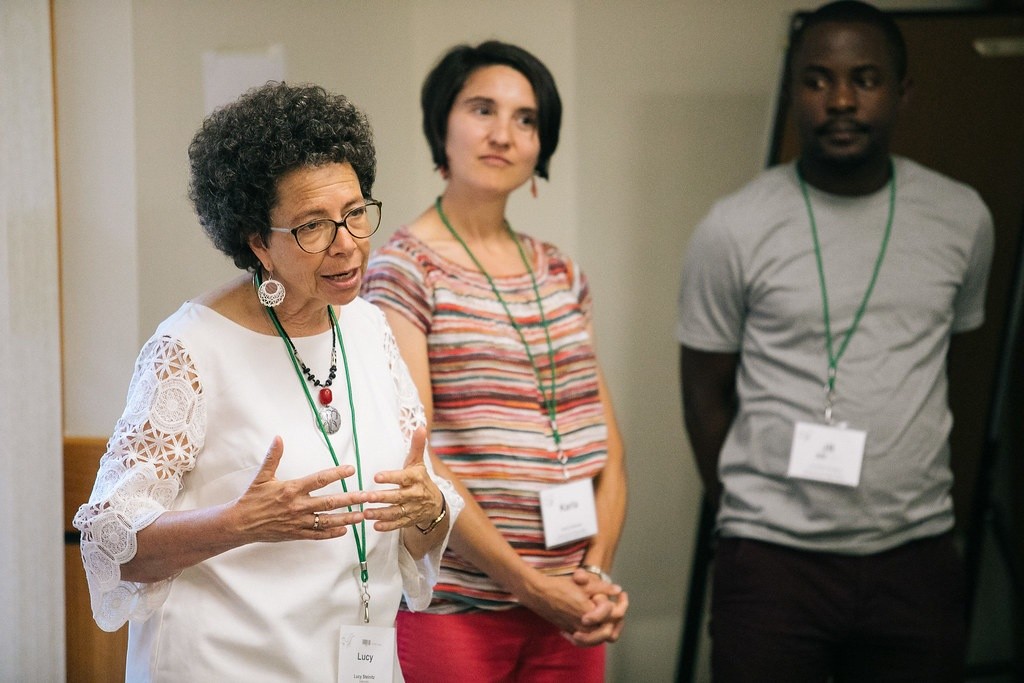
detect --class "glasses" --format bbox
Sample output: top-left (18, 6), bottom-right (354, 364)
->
top-left (270, 198), bottom-right (382, 255)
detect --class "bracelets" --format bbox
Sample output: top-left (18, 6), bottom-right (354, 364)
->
top-left (416, 490), bottom-right (447, 534)
top-left (584, 565), bottom-right (613, 584)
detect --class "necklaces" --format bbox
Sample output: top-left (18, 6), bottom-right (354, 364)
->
top-left (257, 268), bottom-right (340, 434)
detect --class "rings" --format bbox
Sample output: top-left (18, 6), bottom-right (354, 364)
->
top-left (312, 514), bottom-right (320, 531)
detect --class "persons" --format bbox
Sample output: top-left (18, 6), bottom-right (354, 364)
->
top-left (678, 0), bottom-right (996, 683)
top-left (359, 41), bottom-right (628, 683)
top-left (72, 83), bottom-right (463, 683)
